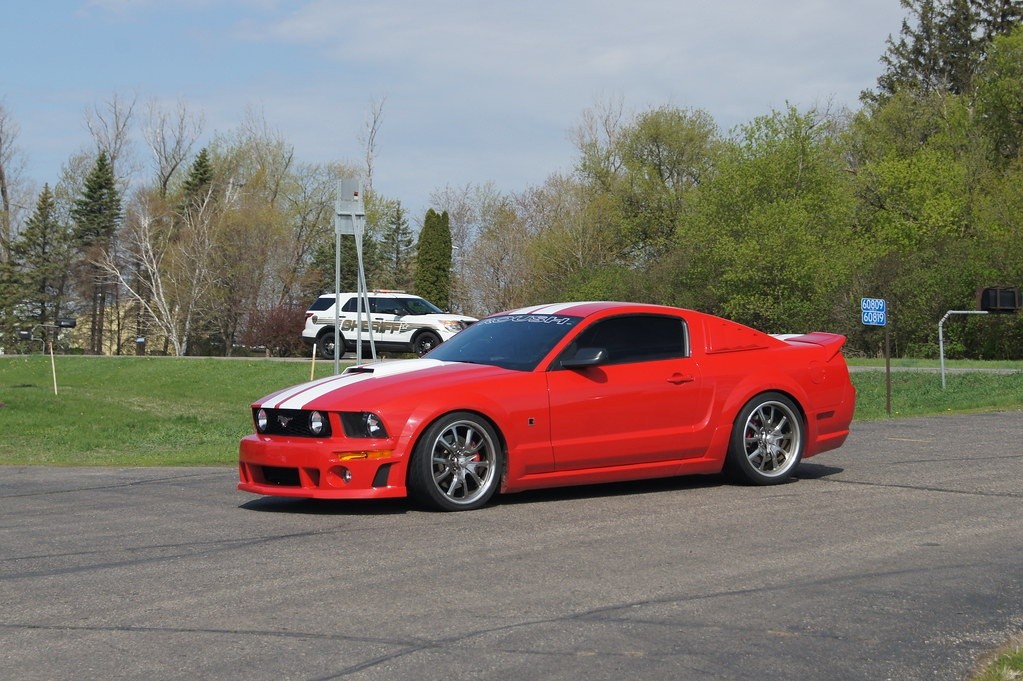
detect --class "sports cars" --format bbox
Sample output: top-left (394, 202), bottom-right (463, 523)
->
top-left (237, 302), bottom-right (856, 511)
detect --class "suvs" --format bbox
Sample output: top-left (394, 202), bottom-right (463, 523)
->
top-left (301, 287), bottom-right (479, 360)
